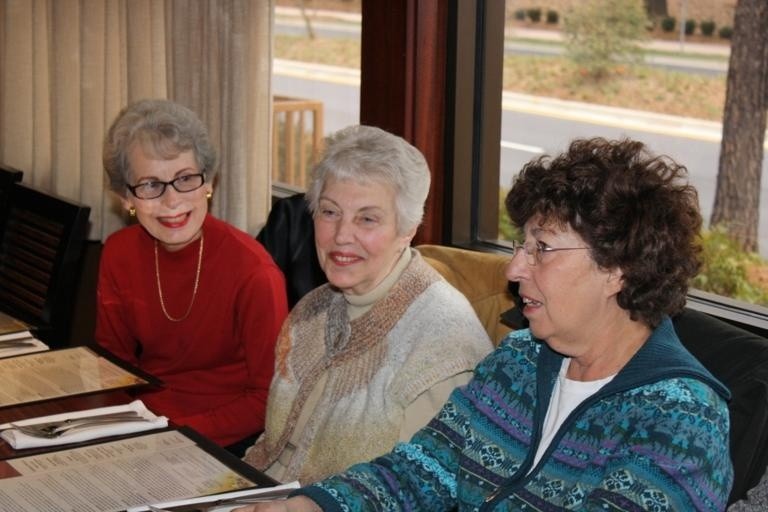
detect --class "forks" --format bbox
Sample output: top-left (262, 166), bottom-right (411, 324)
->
top-left (147, 494), bottom-right (297, 512)
top-left (11, 417), bottom-right (151, 439)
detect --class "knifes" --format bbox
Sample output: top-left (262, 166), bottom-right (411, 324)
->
top-left (137, 489), bottom-right (301, 511)
top-left (0, 412), bottom-right (138, 433)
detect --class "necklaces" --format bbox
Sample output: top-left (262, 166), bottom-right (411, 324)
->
top-left (155, 231), bottom-right (204, 323)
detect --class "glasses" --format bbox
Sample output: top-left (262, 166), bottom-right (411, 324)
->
top-left (125, 169), bottom-right (206, 200)
top-left (512, 239), bottom-right (597, 266)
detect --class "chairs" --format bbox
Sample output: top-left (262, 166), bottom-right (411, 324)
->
top-left (672, 307), bottom-right (768, 511)
top-left (0, 181), bottom-right (91, 350)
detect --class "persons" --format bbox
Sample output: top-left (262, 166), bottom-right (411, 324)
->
top-left (95, 99), bottom-right (289, 448)
top-left (232, 133), bottom-right (735, 512)
top-left (239, 125), bottom-right (496, 488)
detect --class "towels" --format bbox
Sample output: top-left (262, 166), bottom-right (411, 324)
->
top-left (0, 331), bottom-right (50, 359)
top-left (1, 399), bottom-right (169, 449)
top-left (118, 479), bottom-right (302, 512)
